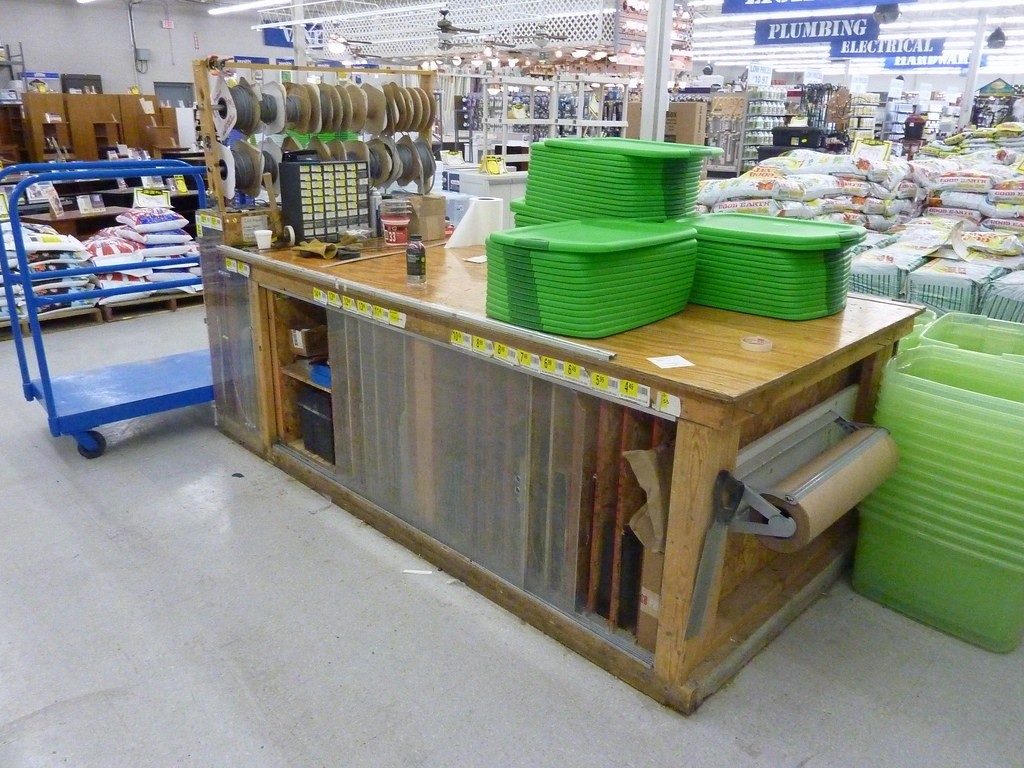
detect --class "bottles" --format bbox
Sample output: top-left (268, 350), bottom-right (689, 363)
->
top-left (744, 87), bottom-right (786, 172)
top-left (406, 236), bottom-right (427, 285)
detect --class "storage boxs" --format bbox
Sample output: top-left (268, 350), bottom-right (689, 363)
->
top-left (626, 102), bottom-right (707, 145)
top-left (287, 323), bottom-right (328, 358)
top-left (850, 312), bottom-right (1024, 654)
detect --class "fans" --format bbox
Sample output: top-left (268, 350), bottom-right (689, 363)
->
top-left (323, 8), bottom-right (566, 61)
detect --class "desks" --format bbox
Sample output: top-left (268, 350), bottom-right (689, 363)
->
top-left (196, 201), bottom-right (926, 717)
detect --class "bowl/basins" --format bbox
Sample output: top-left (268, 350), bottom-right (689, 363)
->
top-left (380, 200), bottom-right (410, 245)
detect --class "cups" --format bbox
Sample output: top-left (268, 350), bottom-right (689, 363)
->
top-left (254, 230), bottom-right (272, 250)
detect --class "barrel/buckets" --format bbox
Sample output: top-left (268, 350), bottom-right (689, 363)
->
top-left (905, 121), bottom-right (926, 139)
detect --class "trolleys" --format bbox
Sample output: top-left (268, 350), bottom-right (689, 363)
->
top-left (0, 160), bottom-right (256, 460)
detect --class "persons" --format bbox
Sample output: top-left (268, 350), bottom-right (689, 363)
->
top-left (970, 101), bottom-right (993, 127)
top-left (997, 107), bottom-right (1019, 126)
top-left (902, 104), bottom-right (926, 161)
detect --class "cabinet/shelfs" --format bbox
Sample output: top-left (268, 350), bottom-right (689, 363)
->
top-left (0, 41), bottom-right (207, 341)
top-left (436, 63), bottom-right (960, 168)
